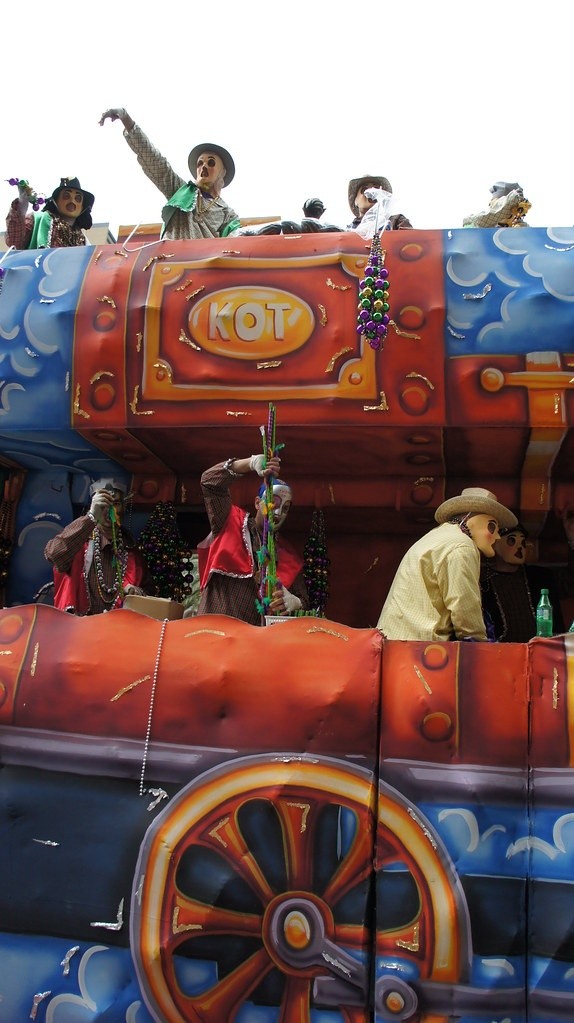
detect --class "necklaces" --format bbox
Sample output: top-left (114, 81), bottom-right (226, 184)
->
top-left (355, 232), bottom-right (390, 353)
top-left (92, 489), bottom-right (129, 604)
top-left (448, 516), bottom-right (473, 540)
top-left (300, 506), bottom-right (331, 619)
top-left (50, 211), bottom-right (86, 247)
top-left (0, 499), bottom-right (14, 608)
top-left (134, 500), bottom-right (195, 604)
top-left (198, 188), bottom-right (218, 215)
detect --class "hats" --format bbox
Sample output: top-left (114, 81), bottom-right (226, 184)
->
top-left (499, 522), bottom-right (529, 537)
top-left (188, 143), bottom-right (234, 188)
top-left (489, 181), bottom-right (523, 202)
top-left (53, 176), bottom-right (95, 211)
top-left (435, 486), bottom-right (517, 530)
top-left (89, 476), bottom-right (128, 499)
top-left (347, 176), bottom-right (393, 217)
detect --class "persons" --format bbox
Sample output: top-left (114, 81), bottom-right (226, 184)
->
top-left (463, 181), bottom-right (532, 227)
top-left (197, 452), bottom-right (309, 627)
top-left (376, 487), bottom-right (567, 641)
top-left (5, 177), bottom-right (95, 250)
top-left (43, 477), bottom-right (143, 617)
top-left (98, 106), bottom-right (243, 241)
top-left (346, 174), bottom-right (413, 231)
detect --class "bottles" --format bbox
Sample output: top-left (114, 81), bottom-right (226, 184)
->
top-left (536, 589), bottom-right (553, 638)
